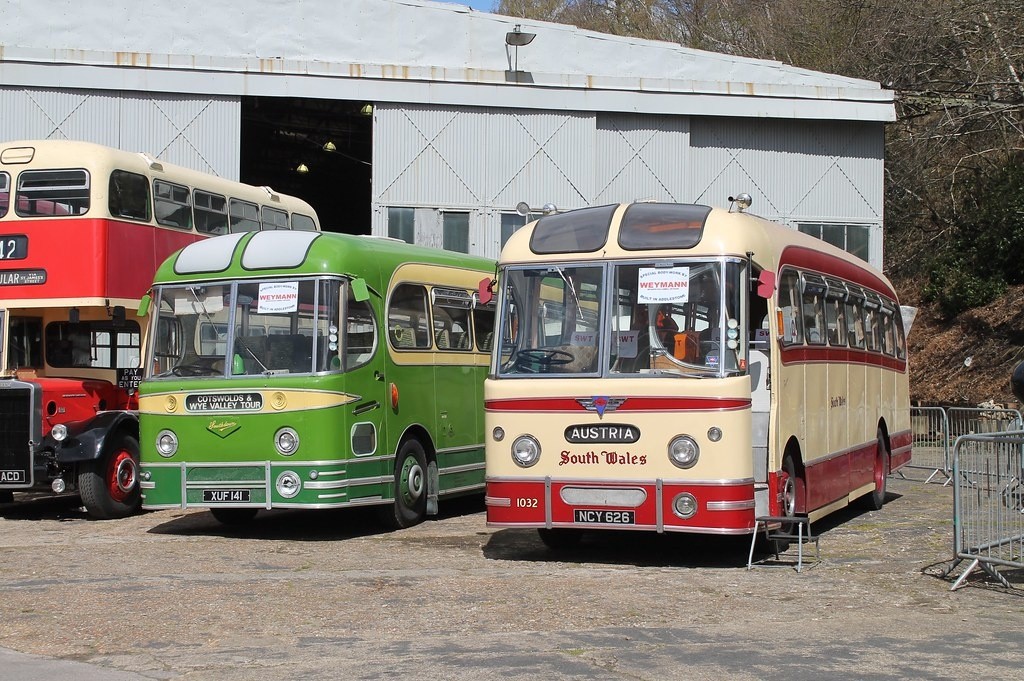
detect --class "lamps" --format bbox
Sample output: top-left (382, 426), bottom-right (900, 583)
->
top-left (360, 104), bottom-right (373, 115)
top-left (297, 164), bottom-right (310, 174)
top-left (323, 141), bottom-right (337, 152)
top-left (508, 24), bottom-right (537, 74)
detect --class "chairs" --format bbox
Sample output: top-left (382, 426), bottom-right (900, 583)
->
top-left (570, 323), bottom-right (874, 380)
top-left (237, 328), bottom-right (494, 375)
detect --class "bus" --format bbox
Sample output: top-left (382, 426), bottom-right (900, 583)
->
top-left (476, 191), bottom-right (916, 552)
top-left (133, 228), bottom-right (601, 528)
top-left (0, 141), bottom-right (321, 520)
